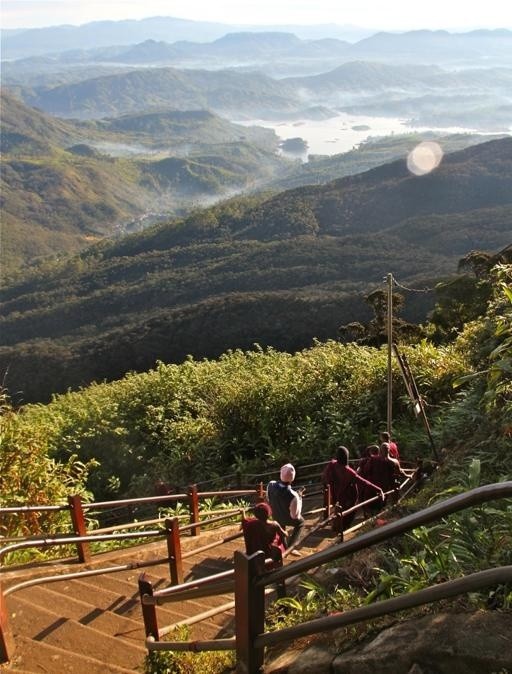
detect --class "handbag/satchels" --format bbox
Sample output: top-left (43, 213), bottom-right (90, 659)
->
top-left (334, 497), bottom-right (351, 511)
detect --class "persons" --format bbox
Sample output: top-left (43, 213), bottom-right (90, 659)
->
top-left (325, 431), bottom-right (414, 532)
top-left (266, 462), bottom-right (305, 557)
top-left (242, 500), bottom-right (290, 598)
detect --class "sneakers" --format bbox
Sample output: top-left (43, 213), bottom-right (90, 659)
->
top-left (290, 549), bottom-right (301, 557)
